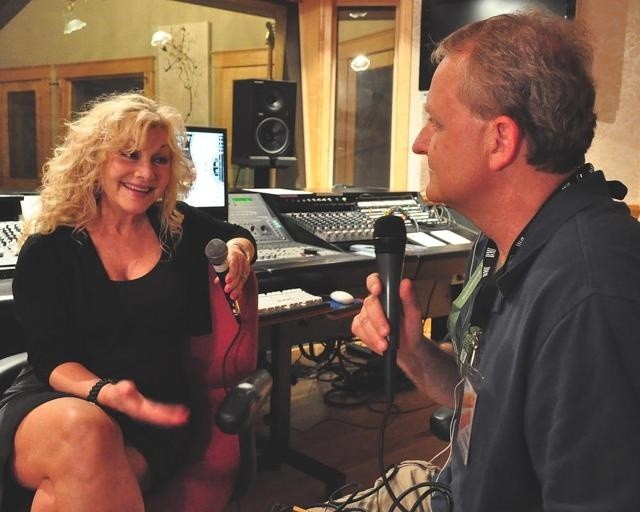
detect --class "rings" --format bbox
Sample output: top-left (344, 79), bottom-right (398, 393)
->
top-left (240, 272), bottom-right (249, 283)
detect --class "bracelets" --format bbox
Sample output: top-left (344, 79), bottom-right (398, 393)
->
top-left (227, 242), bottom-right (250, 261)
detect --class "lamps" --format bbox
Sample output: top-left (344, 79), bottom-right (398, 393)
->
top-left (151, 30), bottom-right (171, 47)
top-left (64, 17), bottom-right (86, 35)
top-left (351, 55), bottom-right (371, 72)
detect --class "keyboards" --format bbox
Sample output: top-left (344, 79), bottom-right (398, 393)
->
top-left (257, 288), bottom-right (323, 316)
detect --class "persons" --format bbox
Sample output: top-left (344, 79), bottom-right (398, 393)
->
top-left (0, 88), bottom-right (257, 512)
top-left (303, 6), bottom-right (640, 512)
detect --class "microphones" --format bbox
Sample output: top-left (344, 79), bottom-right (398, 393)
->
top-left (205, 239), bottom-right (242, 322)
top-left (374, 216), bottom-right (407, 370)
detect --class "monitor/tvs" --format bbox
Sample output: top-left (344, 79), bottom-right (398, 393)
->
top-left (177, 126), bottom-right (228, 221)
top-left (419, 0), bottom-right (576, 90)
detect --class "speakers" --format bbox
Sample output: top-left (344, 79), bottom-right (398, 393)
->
top-left (232, 79), bottom-right (297, 168)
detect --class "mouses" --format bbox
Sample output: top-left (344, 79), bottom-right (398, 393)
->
top-left (330, 291), bottom-right (355, 304)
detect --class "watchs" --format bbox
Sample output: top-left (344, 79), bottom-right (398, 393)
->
top-left (87, 378), bottom-right (119, 404)
